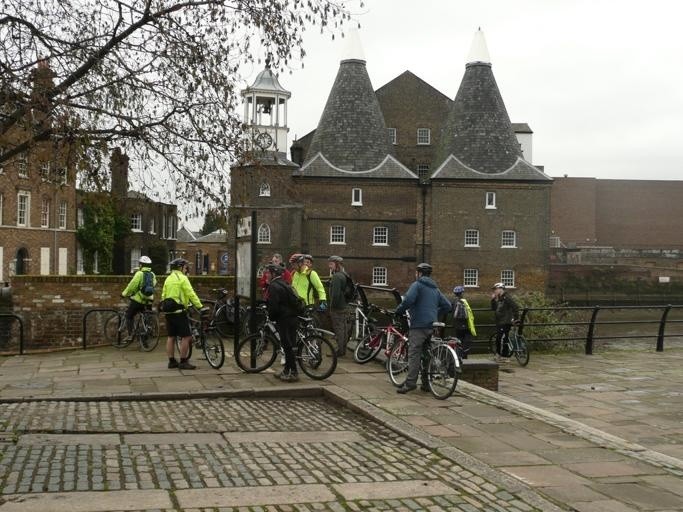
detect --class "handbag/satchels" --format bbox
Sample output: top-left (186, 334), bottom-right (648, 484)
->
top-left (158, 299), bottom-right (184, 313)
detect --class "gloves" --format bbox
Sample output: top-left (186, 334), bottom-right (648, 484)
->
top-left (317, 302), bottom-right (327, 312)
top-left (198, 305), bottom-right (210, 314)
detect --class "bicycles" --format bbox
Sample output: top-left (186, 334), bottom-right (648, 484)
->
top-left (105, 294), bottom-right (161, 352)
top-left (490, 317), bottom-right (530, 368)
top-left (353, 313), bottom-right (413, 374)
top-left (164, 291), bottom-right (340, 382)
top-left (387, 320), bottom-right (462, 399)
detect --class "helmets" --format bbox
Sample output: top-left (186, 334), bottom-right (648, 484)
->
top-left (328, 256), bottom-right (344, 262)
top-left (136, 256), bottom-right (152, 264)
top-left (416, 263), bottom-right (433, 273)
top-left (168, 258), bottom-right (189, 269)
top-left (491, 282), bottom-right (506, 289)
top-left (263, 253), bottom-right (314, 275)
top-left (453, 286), bottom-right (465, 294)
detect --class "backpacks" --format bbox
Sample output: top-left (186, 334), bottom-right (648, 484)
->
top-left (453, 298), bottom-right (467, 320)
top-left (278, 279), bottom-right (305, 310)
top-left (341, 271), bottom-right (358, 302)
top-left (138, 271), bottom-right (154, 296)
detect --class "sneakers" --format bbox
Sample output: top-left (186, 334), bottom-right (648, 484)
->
top-left (274, 367), bottom-right (300, 382)
top-left (421, 383), bottom-right (431, 391)
top-left (489, 354), bottom-right (501, 360)
top-left (178, 362), bottom-right (196, 369)
top-left (326, 349), bottom-right (342, 356)
top-left (123, 335), bottom-right (131, 341)
top-left (396, 383), bottom-right (416, 393)
top-left (167, 359), bottom-right (178, 369)
top-left (496, 356), bottom-right (510, 363)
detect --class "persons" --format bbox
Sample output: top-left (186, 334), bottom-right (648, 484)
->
top-left (119, 256), bottom-right (157, 350)
top-left (451, 286), bottom-right (478, 359)
top-left (256, 251), bottom-right (355, 383)
top-left (160, 258), bottom-right (210, 369)
top-left (489, 282), bottom-right (521, 363)
top-left (393, 263), bottom-right (452, 393)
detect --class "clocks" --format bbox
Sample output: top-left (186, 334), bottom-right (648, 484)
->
top-left (257, 132), bottom-right (273, 149)
